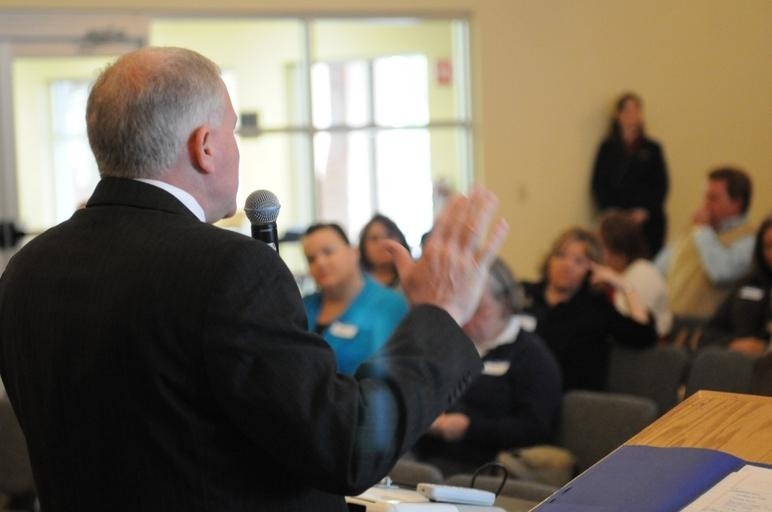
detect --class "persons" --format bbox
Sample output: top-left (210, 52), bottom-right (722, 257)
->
top-left (411, 256), bottom-right (567, 481)
top-left (302, 216), bottom-right (512, 374)
top-left (519, 165), bottom-right (772, 393)
top-left (1, 49), bottom-right (508, 512)
top-left (590, 93), bottom-right (670, 261)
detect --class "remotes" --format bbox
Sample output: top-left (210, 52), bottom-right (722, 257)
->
top-left (417, 483), bottom-right (496, 506)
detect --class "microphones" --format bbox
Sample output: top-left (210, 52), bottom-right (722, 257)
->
top-left (244, 189), bottom-right (281, 256)
